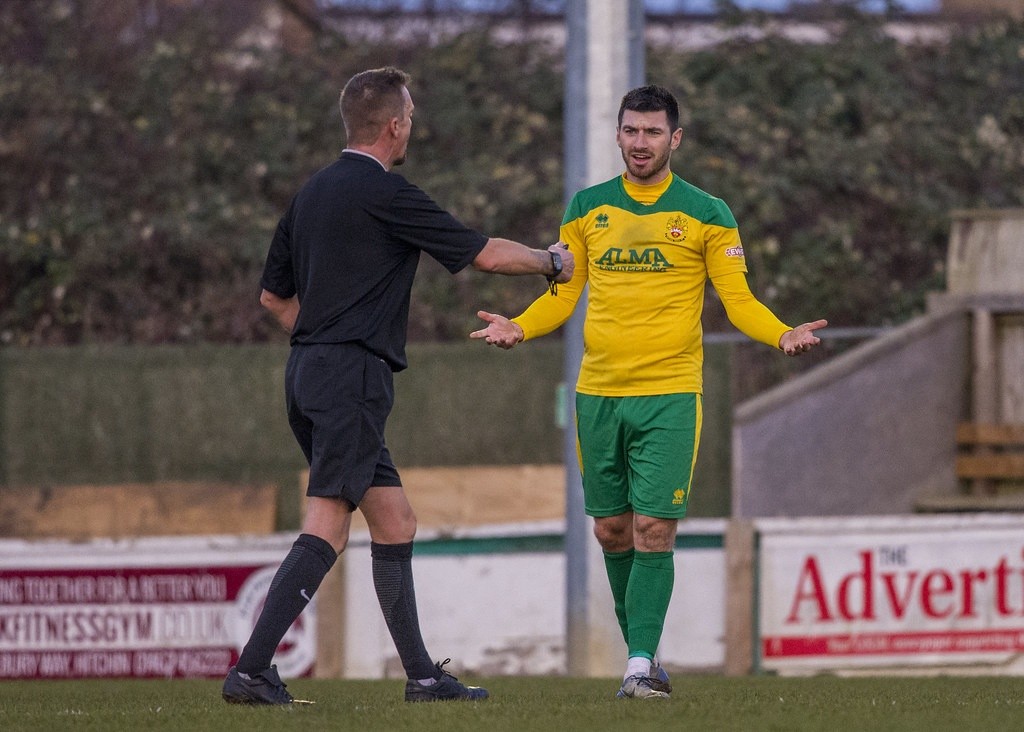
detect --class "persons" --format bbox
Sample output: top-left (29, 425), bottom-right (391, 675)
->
top-left (222, 65), bottom-right (575, 706)
top-left (470, 86), bottom-right (827, 700)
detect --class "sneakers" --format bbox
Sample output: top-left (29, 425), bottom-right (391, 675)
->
top-left (404, 669), bottom-right (491, 704)
top-left (616, 661), bottom-right (671, 706)
top-left (222, 666), bottom-right (315, 705)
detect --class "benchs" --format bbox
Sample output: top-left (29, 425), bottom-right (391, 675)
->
top-left (913, 425), bottom-right (1024, 511)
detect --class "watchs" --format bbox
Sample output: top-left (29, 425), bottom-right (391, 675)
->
top-left (550, 251), bottom-right (564, 279)
